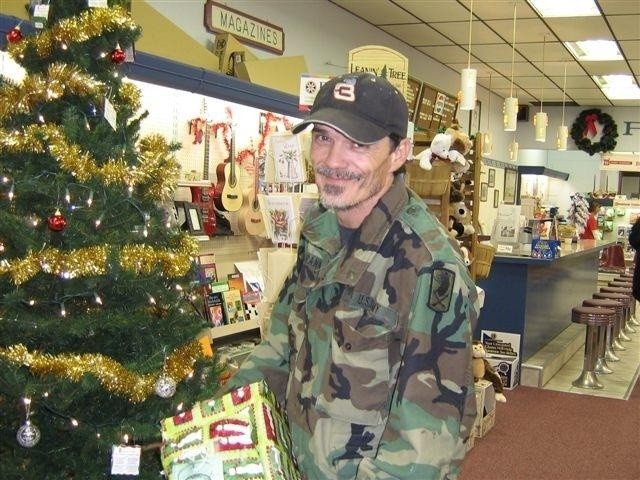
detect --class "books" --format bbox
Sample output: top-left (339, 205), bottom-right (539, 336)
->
top-left (191, 253), bottom-right (262, 327)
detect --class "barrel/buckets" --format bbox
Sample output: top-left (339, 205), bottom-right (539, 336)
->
top-left (459, 242), bottom-right (498, 281)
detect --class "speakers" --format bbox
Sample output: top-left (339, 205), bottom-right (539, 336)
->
top-left (517, 105), bottom-right (529, 122)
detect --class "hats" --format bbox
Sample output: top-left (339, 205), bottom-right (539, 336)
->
top-left (291, 71), bottom-right (409, 146)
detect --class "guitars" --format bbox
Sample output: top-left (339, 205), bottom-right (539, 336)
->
top-left (238, 137), bottom-right (265, 235)
top-left (213, 107), bottom-right (242, 212)
top-left (190, 98), bottom-right (215, 236)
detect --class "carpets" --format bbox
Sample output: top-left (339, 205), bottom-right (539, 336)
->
top-left (448, 386), bottom-right (640, 480)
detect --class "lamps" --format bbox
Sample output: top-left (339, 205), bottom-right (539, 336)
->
top-left (460, 1), bottom-right (567, 163)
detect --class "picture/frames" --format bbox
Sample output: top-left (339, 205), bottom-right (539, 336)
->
top-left (181, 202), bottom-right (204, 235)
top-left (269, 132), bottom-right (306, 183)
top-left (503, 167), bottom-right (518, 204)
top-left (480, 169), bottom-right (498, 208)
top-left (407, 75), bottom-right (458, 138)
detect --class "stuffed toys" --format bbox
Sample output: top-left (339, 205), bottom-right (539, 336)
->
top-left (470, 342), bottom-right (508, 404)
top-left (413, 127), bottom-right (475, 266)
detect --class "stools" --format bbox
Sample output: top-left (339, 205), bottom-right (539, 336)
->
top-left (571, 266), bottom-right (639, 390)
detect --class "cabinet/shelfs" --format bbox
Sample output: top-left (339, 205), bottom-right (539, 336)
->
top-left (406, 132), bottom-right (481, 282)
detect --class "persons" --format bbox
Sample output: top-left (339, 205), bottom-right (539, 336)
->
top-left (628, 216), bottom-right (640, 303)
top-left (581, 204), bottom-right (603, 241)
top-left (210, 70), bottom-right (478, 480)
